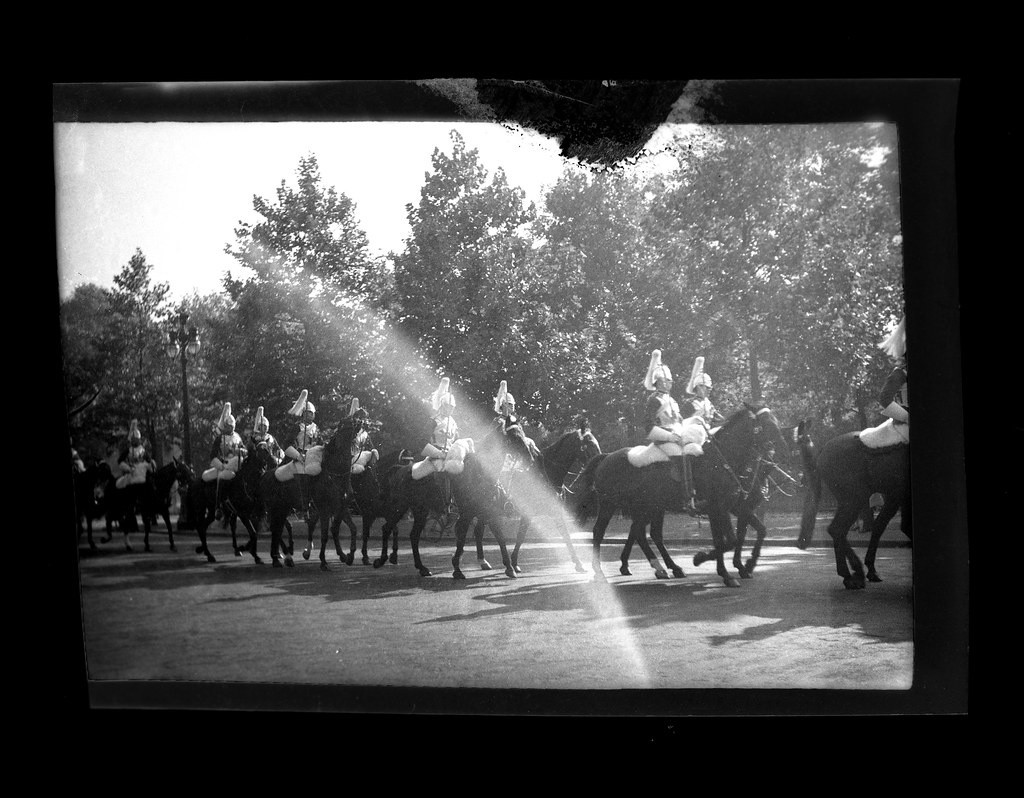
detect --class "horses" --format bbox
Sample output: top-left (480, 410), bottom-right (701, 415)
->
top-left (71, 401), bottom-right (912, 591)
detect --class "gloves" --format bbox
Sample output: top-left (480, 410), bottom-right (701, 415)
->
top-left (75, 460), bottom-right (86, 471)
top-left (421, 443), bottom-right (446, 459)
top-left (210, 457), bottom-right (226, 470)
top-left (284, 445), bottom-right (306, 461)
top-left (119, 462), bottom-right (134, 472)
top-left (647, 426), bottom-right (682, 441)
top-left (880, 402), bottom-right (908, 423)
top-left (682, 416), bottom-right (705, 426)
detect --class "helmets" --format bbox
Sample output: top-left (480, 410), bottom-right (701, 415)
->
top-left (643, 349), bottom-right (673, 390)
top-left (128, 419), bottom-right (140, 441)
top-left (685, 356), bottom-right (712, 393)
top-left (432, 377), bottom-right (455, 410)
top-left (218, 402), bottom-right (236, 431)
top-left (288, 390), bottom-right (316, 417)
top-left (254, 406), bottom-right (269, 432)
top-left (495, 381), bottom-right (516, 413)
top-left (348, 398), bottom-right (369, 422)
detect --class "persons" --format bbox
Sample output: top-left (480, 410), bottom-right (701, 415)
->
top-left (878, 310), bottom-right (909, 426)
top-left (644, 365), bottom-right (715, 509)
top-left (285, 401), bottom-right (324, 514)
top-left (480, 393), bottom-right (541, 495)
top-left (206, 414), bottom-right (247, 500)
top-left (680, 373), bottom-right (726, 438)
top-left (118, 428), bottom-right (157, 472)
top-left (324, 428), bottom-right (380, 478)
top-left (243, 416), bottom-right (282, 497)
top-left (420, 392), bottom-right (472, 523)
